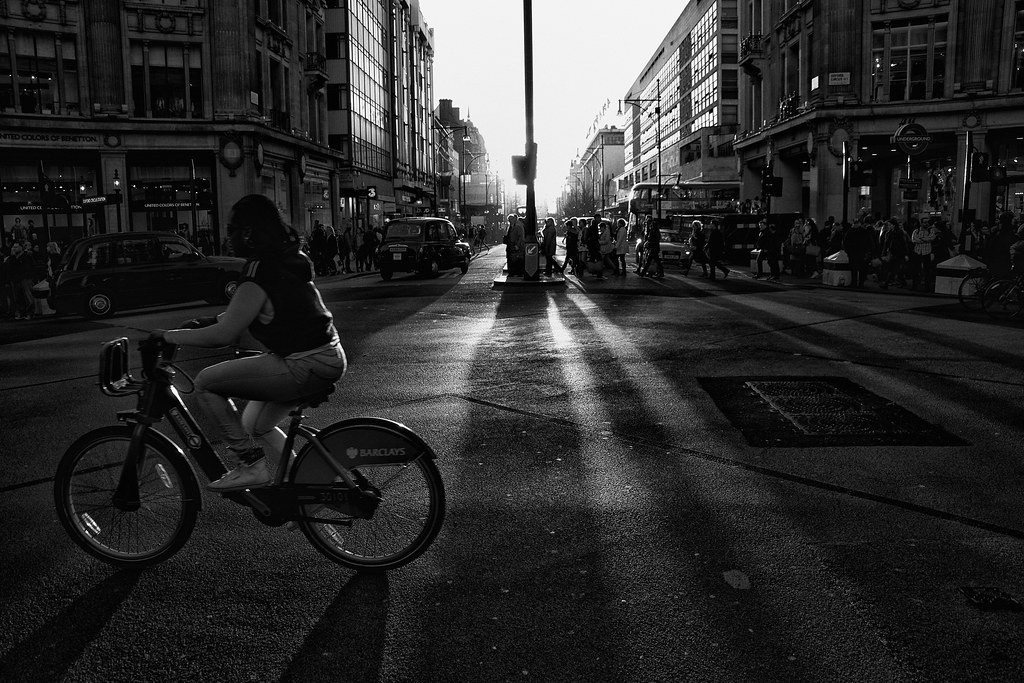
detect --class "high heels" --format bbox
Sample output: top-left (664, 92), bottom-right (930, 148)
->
top-left (811, 272), bottom-right (819, 278)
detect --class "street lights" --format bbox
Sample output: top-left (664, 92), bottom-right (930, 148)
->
top-left (431, 123), bottom-right (472, 218)
top-left (614, 79), bottom-right (665, 225)
top-left (559, 132), bottom-right (609, 220)
top-left (463, 151), bottom-right (490, 215)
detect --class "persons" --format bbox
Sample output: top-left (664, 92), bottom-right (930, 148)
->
top-left (0, 232), bottom-right (63, 320)
top-left (149, 194), bottom-right (347, 531)
top-left (633, 216), bottom-right (665, 279)
top-left (309, 219), bottom-right (386, 277)
top-left (505, 215), bottom-right (526, 276)
top-left (682, 220), bottom-right (730, 280)
top-left (967, 211), bottom-right (1024, 310)
top-left (785, 214), bottom-right (958, 290)
top-left (753, 220), bottom-right (781, 281)
top-left (542, 219), bottom-right (562, 277)
top-left (170, 225), bottom-right (233, 256)
top-left (88, 218), bottom-right (94, 236)
top-left (162, 244), bottom-right (189, 258)
top-left (560, 214), bottom-right (629, 276)
top-left (10, 218), bottom-right (34, 242)
top-left (455, 224), bottom-right (490, 252)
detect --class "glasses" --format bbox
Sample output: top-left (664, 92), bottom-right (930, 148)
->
top-left (226, 224), bottom-right (246, 236)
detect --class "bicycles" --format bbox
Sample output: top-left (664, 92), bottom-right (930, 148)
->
top-left (52, 320), bottom-right (446, 572)
top-left (958, 261), bottom-right (1024, 324)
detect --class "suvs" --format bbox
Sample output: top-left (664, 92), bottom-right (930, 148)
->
top-left (48, 229), bottom-right (251, 322)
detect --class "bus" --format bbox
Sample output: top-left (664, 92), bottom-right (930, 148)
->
top-left (625, 180), bottom-right (741, 240)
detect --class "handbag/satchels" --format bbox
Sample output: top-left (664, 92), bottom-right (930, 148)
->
top-left (805, 241), bottom-right (821, 256)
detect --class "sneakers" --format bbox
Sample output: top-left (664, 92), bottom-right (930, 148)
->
top-left (205, 457), bottom-right (270, 492)
top-left (287, 500), bottom-right (327, 531)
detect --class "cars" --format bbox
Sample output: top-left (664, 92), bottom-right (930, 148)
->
top-left (373, 216), bottom-right (472, 281)
top-left (635, 228), bottom-right (692, 274)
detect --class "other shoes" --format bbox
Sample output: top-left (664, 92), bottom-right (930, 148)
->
top-left (784, 269), bottom-right (792, 274)
top-left (15, 315), bottom-right (23, 320)
top-left (546, 265), bottom-right (627, 279)
top-left (699, 273), bottom-right (707, 277)
top-left (633, 267), bottom-right (664, 278)
top-left (753, 274), bottom-right (761, 278)
top-left (25, 315), bottom-right (31, 320)
top-left (680, 271), bottom-right (688, 276)
top-left (706, 275), bottom-right (715, 279)
top-left (724, 270), bottom-right (729, 279)
